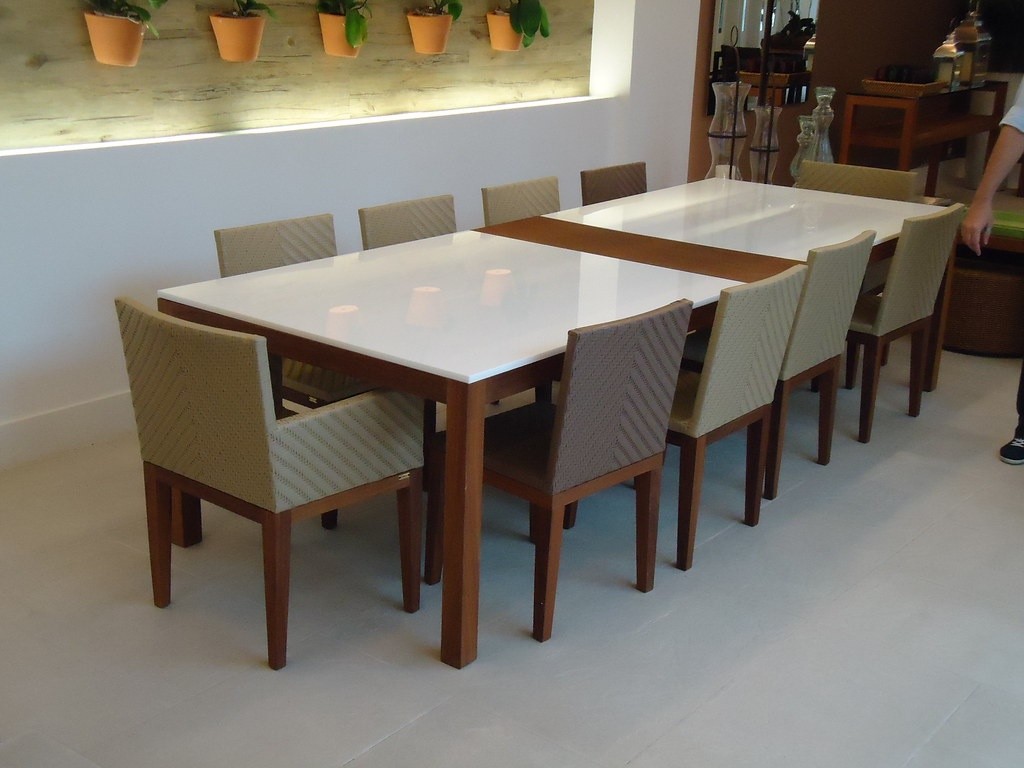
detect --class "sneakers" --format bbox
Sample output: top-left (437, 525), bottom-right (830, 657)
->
top-left (999, 437), bottom-right (1024, 464)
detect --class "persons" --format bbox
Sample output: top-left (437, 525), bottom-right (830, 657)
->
top-left (961, 76), bottom-right (1024, 465)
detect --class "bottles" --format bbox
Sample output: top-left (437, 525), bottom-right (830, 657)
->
top-left (932, 11), bottom-right (993, 94)
top-left (790, 115), bottom-right (815, 181)
top-left (807, 86), bottom-right (836, 163)
top-left (750, 106), bottom-right (782, 183)
top-left (705, 82), bottom-right (751, 181)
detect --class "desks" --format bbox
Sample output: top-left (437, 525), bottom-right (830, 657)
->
top-left (837, 81), bottom-right (1010, 174)
top-left (735, 71), bottom-right (812, 107)
top-left (156, 175), bottom-right (956, 645)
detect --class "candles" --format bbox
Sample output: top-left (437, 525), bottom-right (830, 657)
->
top-left (703, 82), bottom-right (752, 181)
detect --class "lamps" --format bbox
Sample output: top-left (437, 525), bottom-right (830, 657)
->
top-left (933, 32), bottom-right (965, 93)
top-left (951, 0), bottom-right (993, 85)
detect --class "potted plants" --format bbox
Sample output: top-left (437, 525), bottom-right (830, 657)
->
top-left (486, 0), bottom-right (550, 52)
top-left (208, 0), bottom-right (281, 63)
top-left (406, 0), bottom-right (463, 55)
top-left (316, 0), bottom-right (373, 59)
top-left (83, 0), bottom-right (167, 67)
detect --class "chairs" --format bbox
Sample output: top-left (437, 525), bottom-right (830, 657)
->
top-left (212, 213), bottom-right (437, 530)
top-left (480, 175), bottom-right (561, 226)
top-left (423, 298), bottom-right (693, 644)
top-left (562, 262), bottom-right (810, 573)
top-left (113, 294), bottom-right (425, 670)
top-left (581, 161), bottom-right (646, 205)
top-left (814, 200), bottom-right (965, 445)
top-left (357, 193), bottom-right (499, 407)
top-left (796, 159), bottom-right (923, 391)
top-left (680, 229), bottom-right (877, 501)
top-left (722, 45), bottom-right (761, 82)
